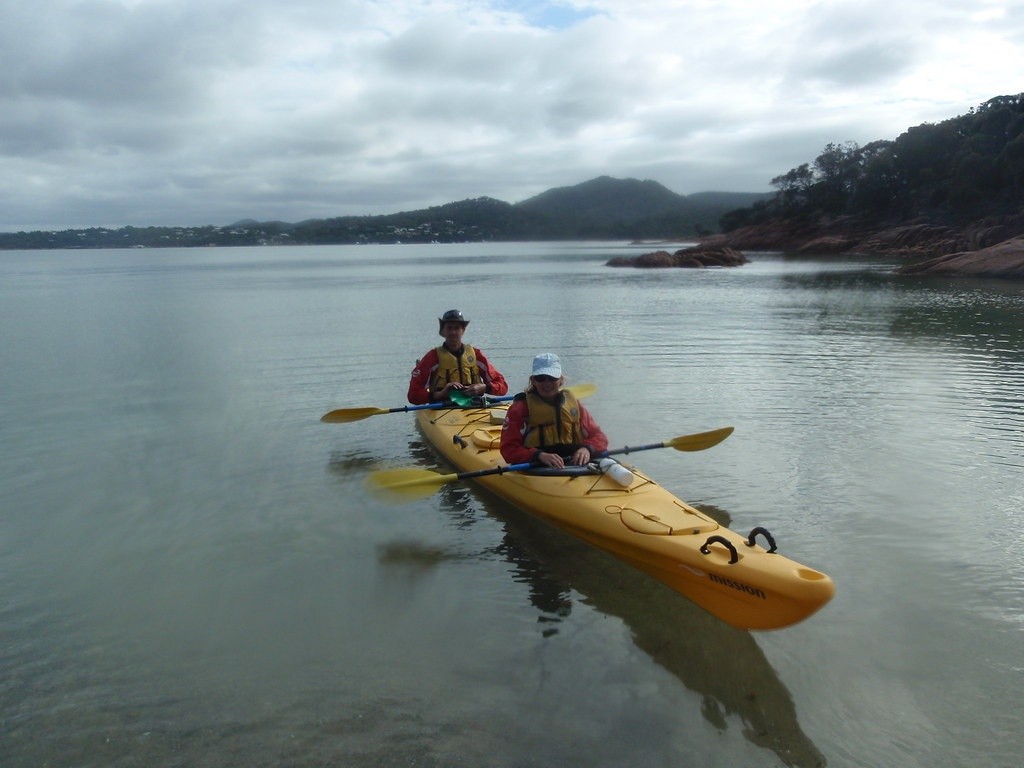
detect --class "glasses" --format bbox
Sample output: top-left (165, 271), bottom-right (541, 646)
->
top-left (532, 375), bottom-right (560, 383)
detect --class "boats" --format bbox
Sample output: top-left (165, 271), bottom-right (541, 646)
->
top-left (412, 395), bottom-right (835, 631)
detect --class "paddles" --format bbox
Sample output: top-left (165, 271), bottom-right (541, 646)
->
top-left (320, 382), bottom-right (597, 424)
top-left (361, 426), bottom-right (736, 507)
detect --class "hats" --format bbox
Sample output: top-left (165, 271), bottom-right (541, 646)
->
top-left (531, 353), bottom-right (562, 378)
top-left (438, 309), bottom-right (470, 337)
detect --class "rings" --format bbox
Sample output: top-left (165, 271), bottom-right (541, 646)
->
top-left (585, 455), bottom-right (588, 458)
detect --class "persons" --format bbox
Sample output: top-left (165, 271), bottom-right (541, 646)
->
top-left (500, 352), bottom-right (609, 469)
top-left (408, 309), bottom-right (508, 410)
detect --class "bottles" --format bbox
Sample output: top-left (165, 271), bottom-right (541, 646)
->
top-left (599, 458), bottom-right (633, 486)
